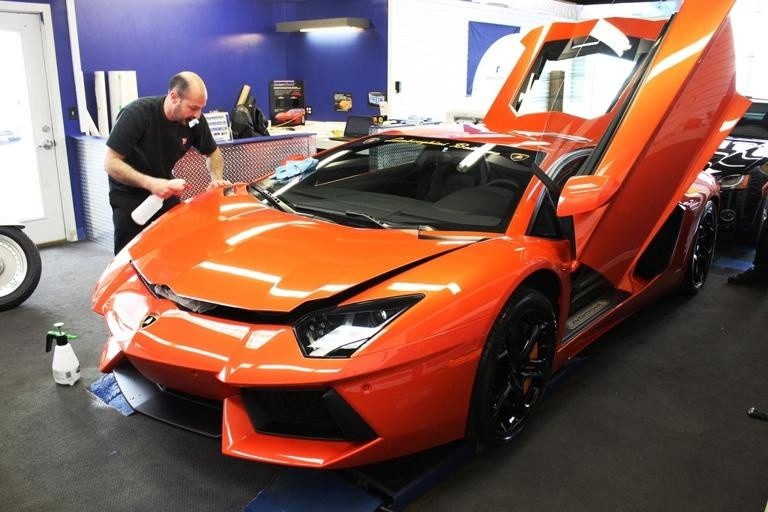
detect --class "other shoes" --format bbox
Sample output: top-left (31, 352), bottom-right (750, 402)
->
top-left (727, 268), bottom-right (760, 285)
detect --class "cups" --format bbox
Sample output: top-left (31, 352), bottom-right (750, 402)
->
top-left (380, 102), bottom-right (391, 115)
top-left (372, 117), bottom-right (378, 123)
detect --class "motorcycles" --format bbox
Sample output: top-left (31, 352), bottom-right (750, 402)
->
top-left (0, 223), bottom-right (43, 312)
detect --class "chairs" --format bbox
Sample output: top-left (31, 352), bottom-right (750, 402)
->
top-left (419, 148), bottom-right (488, 205)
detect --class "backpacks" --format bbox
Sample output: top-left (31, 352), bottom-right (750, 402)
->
top-left (231, 102), bottom-right (270, 139)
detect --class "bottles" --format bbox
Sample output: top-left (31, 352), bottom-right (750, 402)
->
top-left (378, 117), bottom-right (383, 124)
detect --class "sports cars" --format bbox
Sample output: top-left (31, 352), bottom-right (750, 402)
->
top-left (338, 94), bottom-right (351, 109)
top-left (274, 107), bottom-right (304, 124)
top-left (86, 0), bottom-right (752, 473)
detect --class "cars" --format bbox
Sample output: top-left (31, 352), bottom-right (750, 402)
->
top-left (705, 98), bottom-right (767, 246)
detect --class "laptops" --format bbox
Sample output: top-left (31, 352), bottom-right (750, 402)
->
top-left (329, 116), bottom-right (370, 141)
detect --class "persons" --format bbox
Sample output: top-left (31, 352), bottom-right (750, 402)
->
top-left (726, 181), bottom-right (768, 289)
top-left (103, 70), bottom-right (230, 255)
